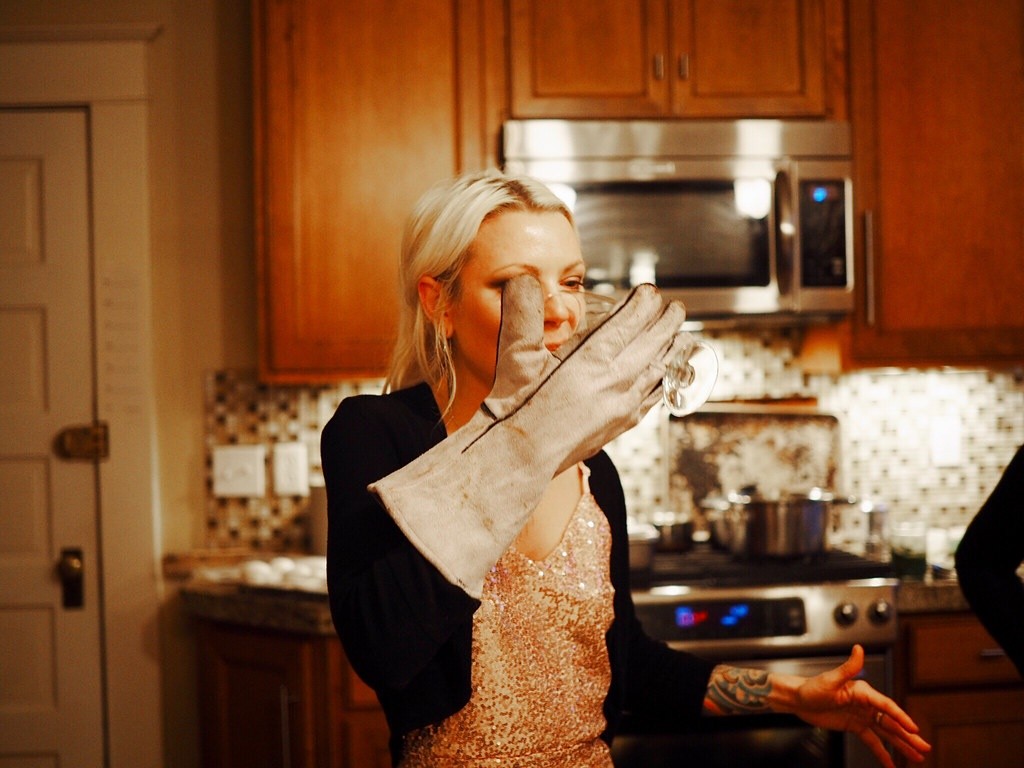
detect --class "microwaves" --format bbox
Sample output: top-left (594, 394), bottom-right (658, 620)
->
top-left (501, 117), bottom-right (856, 315)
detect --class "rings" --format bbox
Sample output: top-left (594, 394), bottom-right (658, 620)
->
top-left (876, 712), bottom-right (883, 724)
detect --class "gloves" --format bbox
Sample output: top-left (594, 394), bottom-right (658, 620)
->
top-left (368, 274), bottom-right (697, 604)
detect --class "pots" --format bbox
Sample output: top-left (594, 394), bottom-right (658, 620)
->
top-left (739, 499), bottom-right (856, 555)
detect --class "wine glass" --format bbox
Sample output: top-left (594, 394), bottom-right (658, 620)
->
top-left (545, 290), bottom-right (719, 418)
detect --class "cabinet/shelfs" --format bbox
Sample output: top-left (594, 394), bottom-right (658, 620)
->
top-left (851, 0), bottom-right (1023, 363)
top-left (251, 1), bottom-right (494, 384)
top-left (194, 629), bottom-right (392, 767)
top-left (899, 608), bottom-right (1024, 767)
top-left (502, 0), bottom-right (850, 118)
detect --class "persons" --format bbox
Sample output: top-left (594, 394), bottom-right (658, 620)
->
top-left (315, 166), bottom-right (933, 768)
top-left (953, 445), bottom-right (1024, 681)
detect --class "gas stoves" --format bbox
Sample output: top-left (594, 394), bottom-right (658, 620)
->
top-left (629, 541), bottom-right (902, 661)
